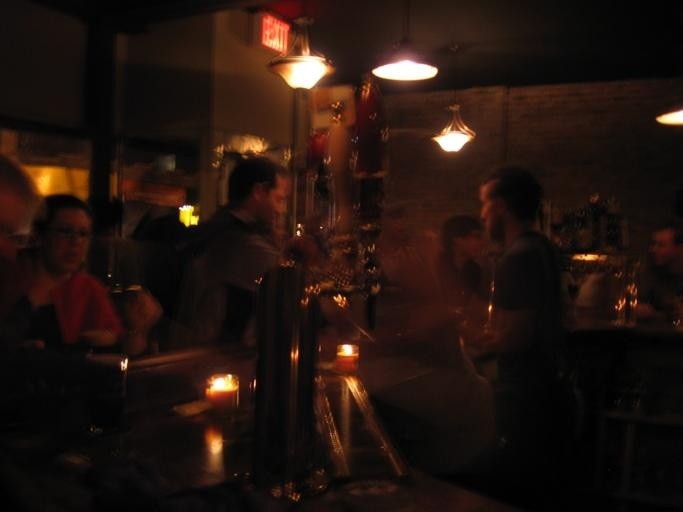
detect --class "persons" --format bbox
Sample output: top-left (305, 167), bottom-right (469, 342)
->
top-left (0, 147), bottom-right (45, 282)
top-left (458, 162), bottom-right (579, 510)
top-left (159, 154), bottom-right (320, 360)
top-left (630, 221), bottom-right (682, 322)
top-left (425, 211), bottom-right (486, 311)
top-left (9, 193), bottom-right (163, 368)
top-left (83, 195), bottom-right (152, 300)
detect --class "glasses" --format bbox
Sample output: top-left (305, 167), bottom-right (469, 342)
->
top-left (0, 224), bottom-right (28, 246)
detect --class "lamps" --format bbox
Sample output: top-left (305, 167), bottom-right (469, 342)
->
top-left (270, 25), bottom-right (477, 162)
top-left (654, 94), bottom-right (683, 126)
